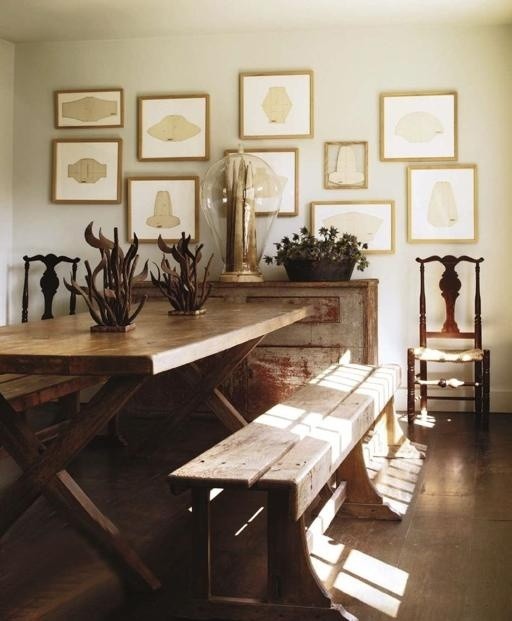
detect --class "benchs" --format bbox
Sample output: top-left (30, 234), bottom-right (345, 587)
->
top-left (1, 366), bottom-right (132, 465)
top-left (160, 361), bottom-right (430, 621)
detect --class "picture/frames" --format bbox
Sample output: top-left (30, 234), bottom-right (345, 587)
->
top-left (123, 173), bottom-right (205, 246)
top-left (404, 163), bottom-right (480, 245)
top-left (307, 201), bottom-right (398, 256)
top-left (322, 140), bottom-right (369, 191)
top-left (236, 69), bottom-right (316, 140)
top-left (219, 146), bottom-right (300, 220)
top-left (373, 90), bottom-right (458, 165)
top-left (134, 92), bottom-right (216, 166)
top-left (49, 135), bottom-right (127, 205)
top-left (52, 88), bottom-right (127, 130)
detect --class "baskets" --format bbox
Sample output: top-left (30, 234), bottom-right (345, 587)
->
top-left (284, 258), bottom-right (357, 280)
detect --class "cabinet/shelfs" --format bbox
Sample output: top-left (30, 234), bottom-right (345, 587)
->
top-left (96, 277), bottom-right (380, 460)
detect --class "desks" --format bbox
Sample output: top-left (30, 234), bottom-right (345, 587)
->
top-left (0, 302), bottom-right (323, 597)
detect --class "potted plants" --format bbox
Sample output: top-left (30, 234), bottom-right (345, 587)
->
top-left (258, 221), bottom-right (371, 285)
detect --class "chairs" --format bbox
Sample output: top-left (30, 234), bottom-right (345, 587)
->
top-left (404, 251), bottom-right (492, 435)
top-left (16, 252), bottom-right (84, 327)
